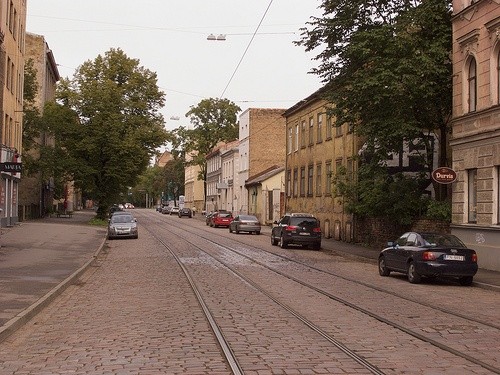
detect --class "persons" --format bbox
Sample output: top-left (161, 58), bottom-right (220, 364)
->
top-left (192, 205), bottom-right (196, 216)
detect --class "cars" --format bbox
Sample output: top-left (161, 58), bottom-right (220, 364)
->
top-left (179, 208), bottom-right (193, 218)
top-left (270, 213), bottom-right (321, 251)
top-left (113, 203), bottom-right (135, 209)
top-left (155, 205), bottom-right (181, 215)
top-left (376, 230), bottom-right (479, 285)
top-left (205, 210), bottom-right (234, 228)
top-left (228, 215), bottom-right (261, 235)
top-left (107, 211), bottom-right (139, 238)
